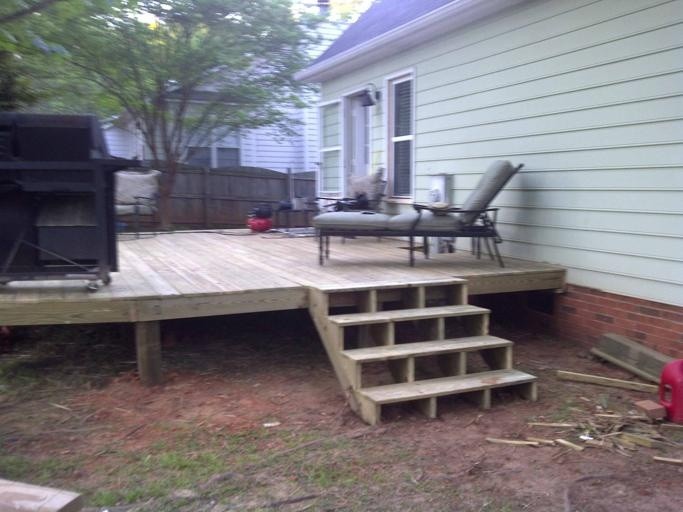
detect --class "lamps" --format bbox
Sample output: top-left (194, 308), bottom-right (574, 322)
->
top-left (359, 83), bottom-right (380, 106)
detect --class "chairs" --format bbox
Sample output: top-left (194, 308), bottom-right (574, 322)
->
top-left (312, 160), bottom-right (524, 267)
top-left (111, 168), bottom-right (162, 239)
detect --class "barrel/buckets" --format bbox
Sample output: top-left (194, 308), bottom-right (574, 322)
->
top-left (657, 357), bottom-right (683, 424)
